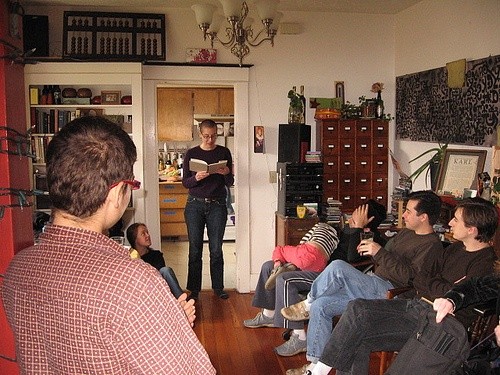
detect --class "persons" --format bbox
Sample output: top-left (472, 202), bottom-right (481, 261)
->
top-left (265, 222), bottom-right (340, 291)
top-left (311, 198), bottom-right (500, 375)
top-left (0, 116), bottom-right (217, 375)
top-left (182, 119), bottom-right (234, 302)
top-left (127, 223), bottom-right (184, 300)
top-left (243, 199), bottom-right (387, 358)
top-left (281, 191), bottom-right (443, 375)
top-left (434, 274), bottom-right (500, 347)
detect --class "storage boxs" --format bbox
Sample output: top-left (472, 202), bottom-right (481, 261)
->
top-left (277, 162), bottom-right (324, 217)
top-left (309, 98), bottom-right (342, 108)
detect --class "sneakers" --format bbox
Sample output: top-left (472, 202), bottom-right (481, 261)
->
top-left (244, 312), bottom-right (275, 328)
top-left (273, 332), bottom-right (307, 356)
top-left (280, 301), bottom-right (310, 321)
top-left (286, 363), bottom-right (310, 375)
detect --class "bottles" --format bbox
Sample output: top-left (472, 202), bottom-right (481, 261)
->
top-left (42, 85), bottom-right (61, 104)
top-left (158, 155), bottom-right (164, 171)
top-left (166, 153), bottom-right (171, 169)
top-left (177, 154), bottom-right (183, 175)
top-left (298, 86), bottom-right (306, 124)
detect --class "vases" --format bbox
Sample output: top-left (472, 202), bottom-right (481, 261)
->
top-left (375, 92), bottom-right (384, 118)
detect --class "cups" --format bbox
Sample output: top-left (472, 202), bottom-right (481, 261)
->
top-left (360, 232), bottom-right (375, 257)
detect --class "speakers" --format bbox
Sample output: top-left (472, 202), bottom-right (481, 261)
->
top-left (278, 124), bottom-right (312, 163)
top-left (21, 14), bottom-right (50, 57)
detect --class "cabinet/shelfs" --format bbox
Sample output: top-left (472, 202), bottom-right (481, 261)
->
top-left (315, 118), bottom-right (390, 213)
top-left (24, 61), bottom-right (146, 247)
top-left (274, 213), bottom-right (399, 270)
top-left (159, 184), bottom-right (190, 236)
top-left (156, 87), bottom-right (234, 141)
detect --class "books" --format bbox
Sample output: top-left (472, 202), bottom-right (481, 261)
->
top-left (31, 108), bottom-right (87, 163)
top-left (189, 159), bottom-right (227, 174)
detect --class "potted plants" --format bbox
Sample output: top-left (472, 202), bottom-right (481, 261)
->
top-left (404, 117), bottom-right (466, 193)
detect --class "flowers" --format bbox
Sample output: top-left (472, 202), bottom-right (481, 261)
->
top-left (372, 83), bottom-right (384, 93)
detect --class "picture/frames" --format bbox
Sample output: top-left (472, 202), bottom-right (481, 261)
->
top-left (435, 149), bottom-right (487, 198)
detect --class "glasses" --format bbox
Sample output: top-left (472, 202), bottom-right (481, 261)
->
top-left (108, 180), bottom-right (141, 190)
top-left (201, 131), bottom-right (218, 139)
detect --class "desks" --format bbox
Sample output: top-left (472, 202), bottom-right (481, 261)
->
top-left (438, 194), bottom-right (462, 229)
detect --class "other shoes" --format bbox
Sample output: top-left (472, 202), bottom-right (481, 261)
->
top-left (188, 291), bottom-right (198, 299)
top-left (214, 289), bottom-right (228, 300)
top-left (265, 263), bottom-right (297, 291)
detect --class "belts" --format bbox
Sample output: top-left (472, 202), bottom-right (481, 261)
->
top-left (188, 192), bottom-right (226, 203)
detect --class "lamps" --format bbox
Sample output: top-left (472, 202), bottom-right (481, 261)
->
top-left (191, 0), bottom-right (284, 68)
